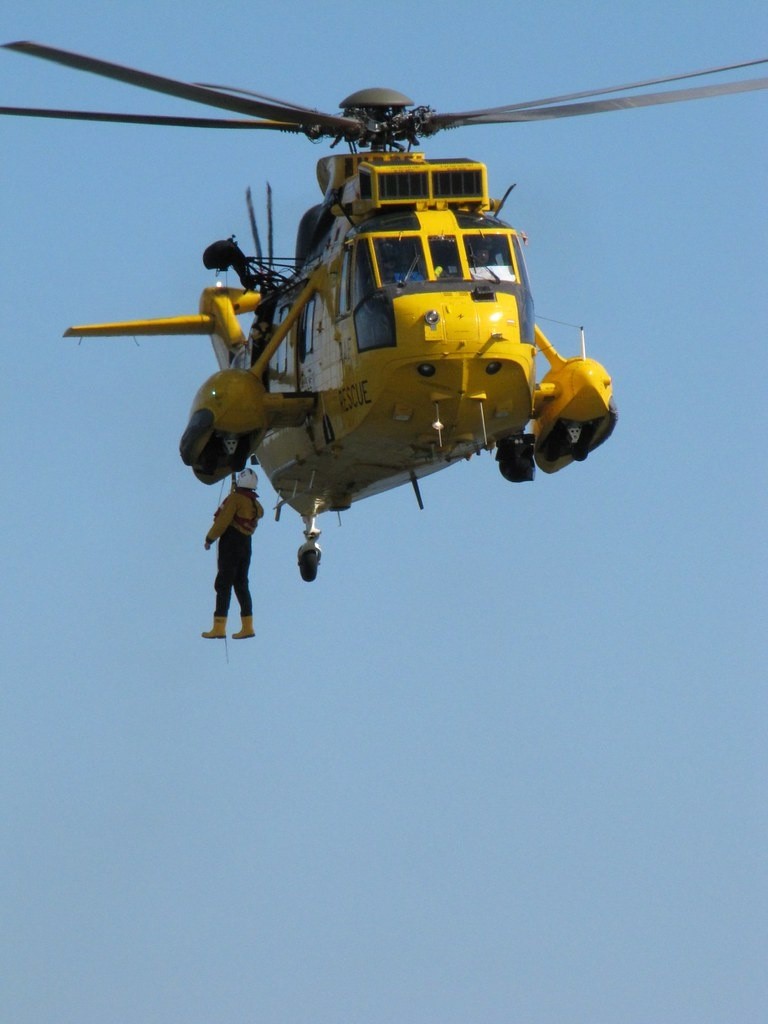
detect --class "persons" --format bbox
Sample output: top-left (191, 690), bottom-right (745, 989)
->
top-left (470, 240), bottom-right (495, 267)
top-left (200, 467), bottom-right (264, 639)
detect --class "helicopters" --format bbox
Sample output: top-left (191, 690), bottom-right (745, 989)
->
top-left (1, 36), bottom-right (767, 583)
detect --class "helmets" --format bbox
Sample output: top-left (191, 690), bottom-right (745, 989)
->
top-left (236, 468), bottom-right (258, 490)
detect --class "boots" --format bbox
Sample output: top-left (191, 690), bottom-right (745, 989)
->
top-left (232, 615), bottom-right (256, 639)
top-left (201, 616), bottom-right (228, 639)
